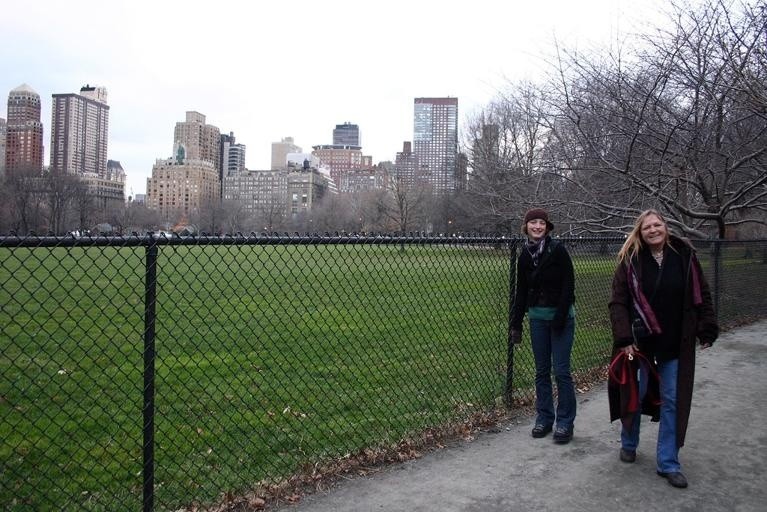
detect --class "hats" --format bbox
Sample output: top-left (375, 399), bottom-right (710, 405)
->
top-left (525, 209), bottom-right (554, 230)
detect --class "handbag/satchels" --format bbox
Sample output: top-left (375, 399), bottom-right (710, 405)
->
top-left (632, 319), bottom-right (652, 359)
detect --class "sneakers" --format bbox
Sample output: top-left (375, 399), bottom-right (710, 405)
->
top-left (657, 470), bottom-right (688, 487)
top-left (621, 449), bottom-right (636, 462)
top-left (532, 423), bottom-right (552, 438)
top-left (553, 426), bottom-right (573, 441)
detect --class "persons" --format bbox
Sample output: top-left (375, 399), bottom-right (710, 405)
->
top-left (508, 209), bottom-right (576, 444)
top-left (608, 210), bottom-right (719, 489)
